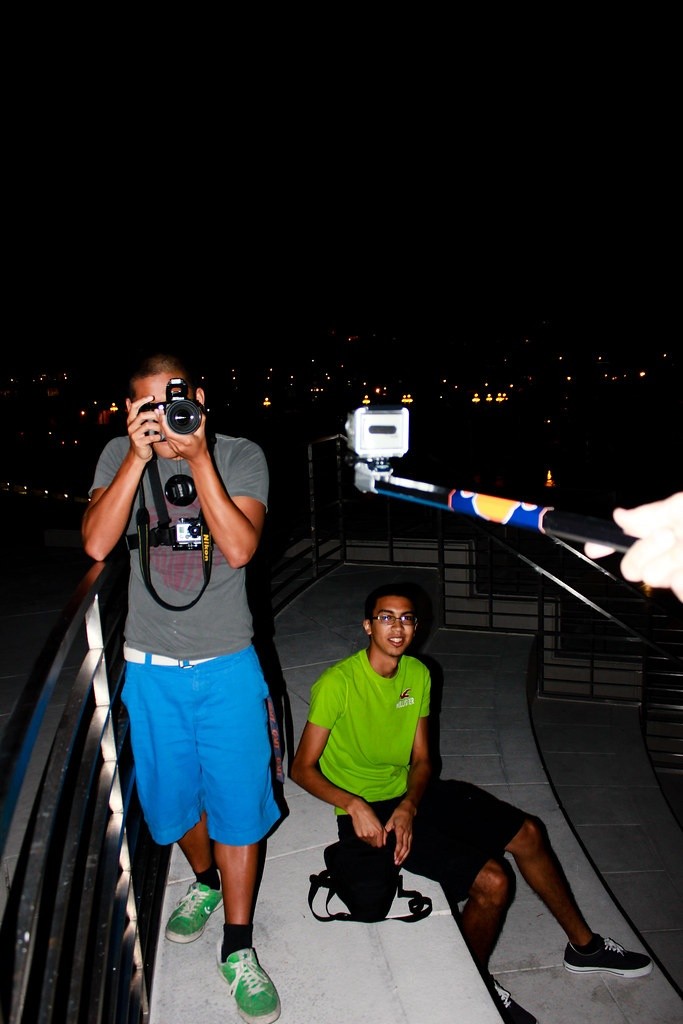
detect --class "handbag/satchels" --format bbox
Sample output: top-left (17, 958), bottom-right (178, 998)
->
top-left (308, 829), bottom-right (434, 922)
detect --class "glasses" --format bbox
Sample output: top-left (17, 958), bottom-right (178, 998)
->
top-left (372, 614), bottom-right (417, 626)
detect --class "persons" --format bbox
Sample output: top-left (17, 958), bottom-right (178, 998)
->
top-left (83, 356), bottom-right (291, 1023)
top-left (584, 490), bottom-right (682, 606)
top-left (288, 584), bottom-right (654, 1024)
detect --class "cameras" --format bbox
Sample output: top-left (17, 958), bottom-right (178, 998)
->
top-left (345, 406), bottom-right (410, 470)
top-left (171, 518), bottom-right (204, 551)
top-left (137, 378), bottom-right (201, 443)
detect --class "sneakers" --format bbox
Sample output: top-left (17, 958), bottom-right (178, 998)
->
top-left (563, 934), bottom-right (654, 978)
top-left (485, 974), bottom-right (540, 1024)
top-left (165, 870), bottom-right (282, 1024)
